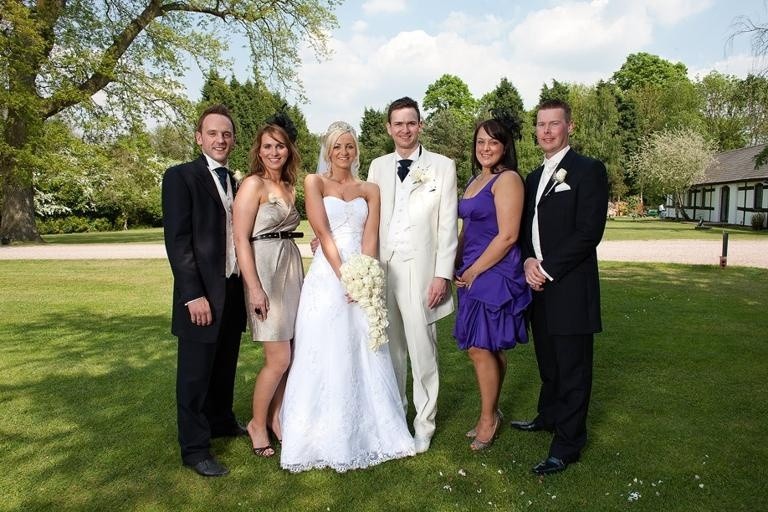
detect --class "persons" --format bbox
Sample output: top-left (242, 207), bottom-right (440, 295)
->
top-left (162, 106), bottom-right (249, 476)
top-left (366, 98), bottom-right (459, 456)
top-left (233, 122), bottom-right (306, 458)
top-left (512, 100), bottom-right (609, 477)
top-left (456, 119), bottom-right (533, 451)
top-left (280, 120), bottom-right (418, 475)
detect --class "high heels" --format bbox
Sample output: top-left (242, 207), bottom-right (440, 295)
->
top-left (466, 409), bottom-right (504, 450)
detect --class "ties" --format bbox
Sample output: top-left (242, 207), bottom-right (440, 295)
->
top-left (542, 162), bottom-right (556, 186)
top-left (397, 159), bottom-right (413, 182)
top-left (215, 167), bottom-right (227, 195)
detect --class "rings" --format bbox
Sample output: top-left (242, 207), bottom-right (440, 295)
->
top-left (254, 307), bottom-right (260, 315)
top-left (196, 318), bottom-right (202, 321)
top-left (438, 297), bottom-right (443, 302)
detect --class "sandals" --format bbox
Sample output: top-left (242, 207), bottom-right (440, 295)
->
top-left (246, 427), bottom-right (281, 458)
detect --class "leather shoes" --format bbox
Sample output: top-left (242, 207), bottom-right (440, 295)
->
top-left (533, 456), bottom-right (579, 473)
top-left (510, 421), bottom-right (555, 433)
top-left (183, 457), bottom-right (230, 477)
top-left (211, 422), bottom-right (249, 438)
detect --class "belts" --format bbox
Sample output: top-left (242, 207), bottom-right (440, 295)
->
top-left (249, 231), bottom-right (303, 242)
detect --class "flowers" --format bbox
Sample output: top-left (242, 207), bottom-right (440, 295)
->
top-left (409, 165), bottom-right (431, 185)
top-left (544, 168), bottom-right (568, 196)
top-left (339, 254), bottom-right (390, 353)
